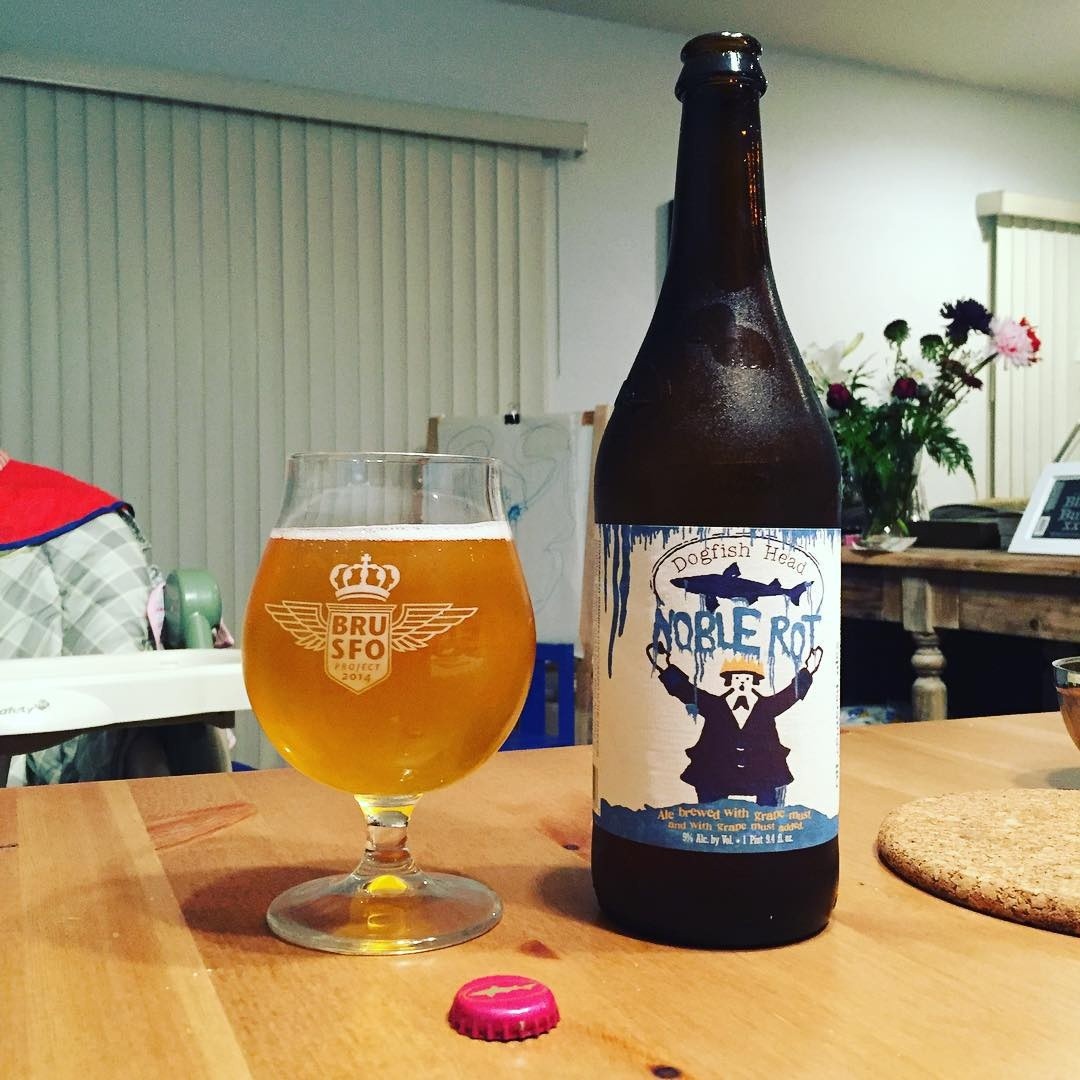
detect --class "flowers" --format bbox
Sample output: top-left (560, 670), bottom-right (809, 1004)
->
top-left (799, 296), bottom-right (1043, 538)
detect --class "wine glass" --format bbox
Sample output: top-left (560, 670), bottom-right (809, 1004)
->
top-left (244, 456), bottom-right (542, 956)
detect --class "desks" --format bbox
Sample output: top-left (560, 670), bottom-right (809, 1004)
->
top-left (0, 709), bottom-right (1080, 1080)
top-left (841, 542), bottom-right (1079, 723)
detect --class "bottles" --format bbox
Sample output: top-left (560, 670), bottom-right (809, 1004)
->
top-left (586, 29), bottom-right (844, 952)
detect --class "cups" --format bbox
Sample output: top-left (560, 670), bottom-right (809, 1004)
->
top-left (1052, 656), bottom-right (1080, 756)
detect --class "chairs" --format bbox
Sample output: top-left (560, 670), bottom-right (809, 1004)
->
top-left (0, 566), bottom-right (251, 791)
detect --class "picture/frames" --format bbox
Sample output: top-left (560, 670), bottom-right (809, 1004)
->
top-left (1007, 463), bottom-right (1080, 557)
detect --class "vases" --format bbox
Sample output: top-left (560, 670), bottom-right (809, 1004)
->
top-left (839, 440), bottom-right (930, 547)
